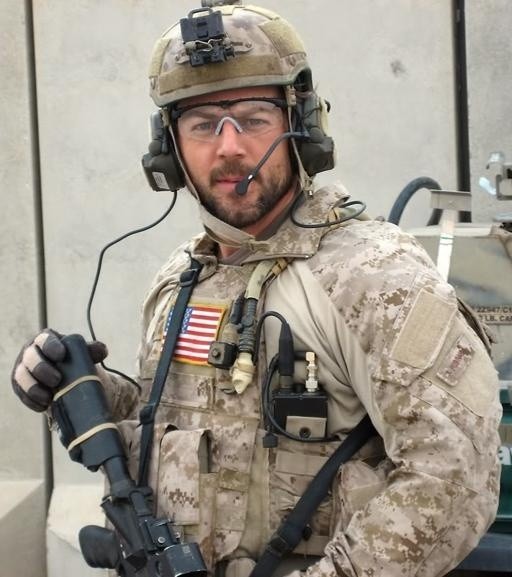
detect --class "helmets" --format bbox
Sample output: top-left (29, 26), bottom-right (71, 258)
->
top-left (149, 4), bottom-right (313, 107)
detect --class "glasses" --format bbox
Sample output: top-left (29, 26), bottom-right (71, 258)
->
top-left (170, 97), bottom-right (290, 142)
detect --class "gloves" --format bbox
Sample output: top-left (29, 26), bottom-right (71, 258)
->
top-left (11, 329), bottom-right (117, 412)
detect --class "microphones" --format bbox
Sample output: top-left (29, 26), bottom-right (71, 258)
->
top-left (235, 130), bottom-right (311, 197)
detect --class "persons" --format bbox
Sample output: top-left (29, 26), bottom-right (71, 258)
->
top-left (10, 3), bottom-right (504, 577)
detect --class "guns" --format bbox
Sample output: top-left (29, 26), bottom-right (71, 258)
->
top-left (49, 334), bottom-right (210, 577)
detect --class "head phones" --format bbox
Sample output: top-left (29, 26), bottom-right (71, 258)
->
top-left (141, 90), bottom-right (335, 192)
